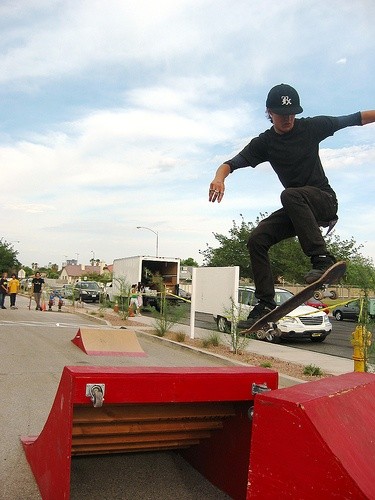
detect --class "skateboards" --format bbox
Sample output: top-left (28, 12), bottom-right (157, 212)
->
top-left (27, 295), bottom-right (32, 310)
top-left (240, 261), bottom-right (349, 335)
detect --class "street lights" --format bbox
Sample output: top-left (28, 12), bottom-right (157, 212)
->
top-left (91, 251), bottom-right (95, 258)
top-left (137, 226), bottom-right (159, 256)
top-left (75, 253), bottom-right (79, 260)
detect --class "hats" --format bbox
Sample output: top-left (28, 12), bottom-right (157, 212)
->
top-left (266, 83), bottom-right (304, 115)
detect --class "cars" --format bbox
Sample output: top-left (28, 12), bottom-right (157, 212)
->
top-left (59, 284), bottom-right (73, 300)
top-left (19, 278), bottom-right (33, 290)
top-left (179, 288), bottom-right (191, 302)
top-left (304, 298), bottom-right (329, 314)
top-left (74, 281), bottom-right (103, 301)
top-left (333, 297), bottom-right (375, 323)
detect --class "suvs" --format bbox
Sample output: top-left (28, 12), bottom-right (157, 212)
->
top-left (212, 286), bottom-right (332, 342)
top-left (314, 289), bottom-right (337, 301)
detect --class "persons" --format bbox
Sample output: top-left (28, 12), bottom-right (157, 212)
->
top-left (208, 82), bottom-right (375, 340)
top-left (127, 285), bottom-right (142, 317)
top-left (6, 273), bottom-right (21, 309)
top-left (31, 272), bottom-right (46, 311)
top-left (0, 272), bottom-right (9, 309)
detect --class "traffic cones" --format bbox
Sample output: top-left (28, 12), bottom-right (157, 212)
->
top-left (114, 299), bottom-right (119, 312)
top-left (127, 305), bottom-right (135, 317)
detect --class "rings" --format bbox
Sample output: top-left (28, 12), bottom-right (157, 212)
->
top-left (210, 188), bottom-right (225, 195)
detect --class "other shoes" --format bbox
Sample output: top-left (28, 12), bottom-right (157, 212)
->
top-left (304, 257), bottom-right (334, 284)
top-left (237, 304), bottom-right (271, 328)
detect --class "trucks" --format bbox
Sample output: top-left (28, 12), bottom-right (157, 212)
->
top-left (103, 256), bottom-right (180, 313)
